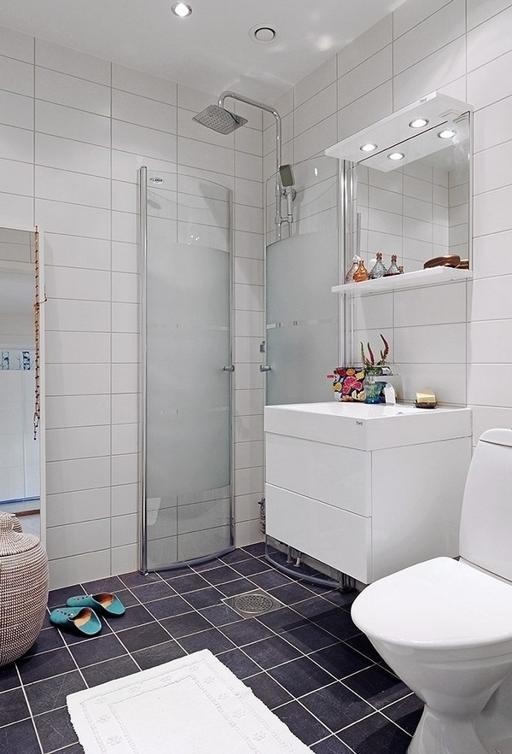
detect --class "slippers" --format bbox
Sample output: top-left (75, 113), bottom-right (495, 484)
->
top-left (49, 606), bottom-right (102, 636)
top-left (65, 592), bottom-right (125, 615)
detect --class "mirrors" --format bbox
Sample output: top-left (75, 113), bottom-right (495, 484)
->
top-left (335, 111), bottom-right (471, 407)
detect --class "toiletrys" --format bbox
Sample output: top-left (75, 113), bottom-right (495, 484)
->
top-left (344, 261), bottom-right (358, 284)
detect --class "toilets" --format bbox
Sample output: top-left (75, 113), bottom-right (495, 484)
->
top-left (350, 427), bottom-right (512, 753)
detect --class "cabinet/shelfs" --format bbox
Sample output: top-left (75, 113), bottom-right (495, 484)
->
top-left (262, 400), bottom-right (474, 581)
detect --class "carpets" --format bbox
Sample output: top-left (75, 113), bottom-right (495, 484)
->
top-left (65, 647), bottom-right (316, 754)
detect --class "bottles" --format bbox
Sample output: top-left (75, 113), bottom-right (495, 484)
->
top-left (345, 255), bottom-right (359, 283)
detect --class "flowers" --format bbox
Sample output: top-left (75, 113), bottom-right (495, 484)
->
top-left (325, 333), bottom-right (391, 404)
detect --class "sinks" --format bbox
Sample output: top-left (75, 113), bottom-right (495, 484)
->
top-left (262, 398), bottom-right (471, 420)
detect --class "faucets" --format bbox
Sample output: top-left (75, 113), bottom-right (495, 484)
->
top-left (366, 364), bottom-right (403, 404)
